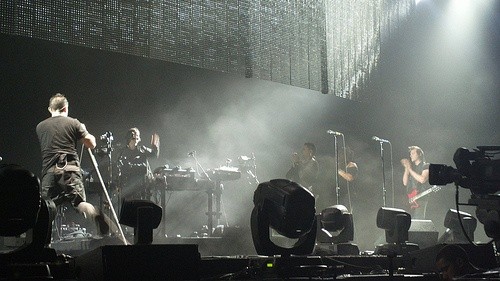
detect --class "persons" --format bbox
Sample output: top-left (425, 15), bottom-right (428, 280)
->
top-left (287, 142), bottom-right (358, 210)
top-left (434, 244), bottom-right (481, 281)
top-left (117, 127), bottom-right (160, 204)
top-left (400, 146), bottom-right (432, 218)
top-left (36, 93), bottom-right (108, 247)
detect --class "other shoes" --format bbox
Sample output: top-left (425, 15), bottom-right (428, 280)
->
top-left (92, 213), bottom-right (109, 235)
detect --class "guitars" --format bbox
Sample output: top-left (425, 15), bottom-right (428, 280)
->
top-left (407, 185), bottom-right (444, 209)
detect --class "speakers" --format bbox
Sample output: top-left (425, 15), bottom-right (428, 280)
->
top-left (407, 219), bottom-right (438, 248)
top-left (69, 243), bottom-right (201, 281)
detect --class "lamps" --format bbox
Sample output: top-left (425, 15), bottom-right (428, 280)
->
top-left (315, 205), bottom-right (355, 243)
top-left (376, 207), bottom-right (411, 243)
top-left (119, 200), bottom-right (165, 243)
top-left (444, 208), bottom-right (478, 242)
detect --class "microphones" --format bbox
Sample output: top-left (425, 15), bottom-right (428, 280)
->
top-left (325, 129), bottom-right (342, 137)
top-left (371, 135), bottom-right (388, 142)
top-left (187, 150), bottom-right (194, 157)
